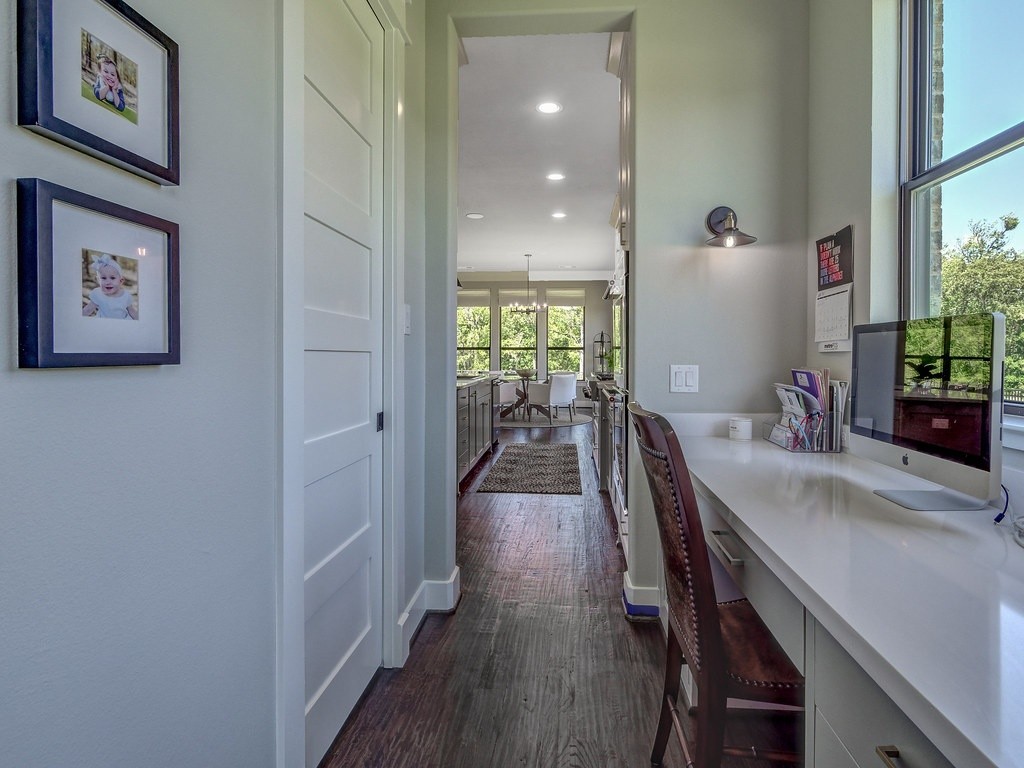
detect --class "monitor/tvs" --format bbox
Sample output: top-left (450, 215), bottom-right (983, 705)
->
top-left (849, 313), bottom-right (1006, 510)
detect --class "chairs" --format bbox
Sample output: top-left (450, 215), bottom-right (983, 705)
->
top-left (528, 371), bottom-right (578, 425)
top-left (627, 401), bottom-right (807, 768)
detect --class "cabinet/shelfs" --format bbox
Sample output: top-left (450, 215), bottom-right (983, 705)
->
top-left (457, 373), bottom-right (501, 497)
top-left (647, 412), bottom-right (1024, 768)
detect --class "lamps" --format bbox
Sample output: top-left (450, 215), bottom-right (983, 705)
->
top-left (510, 253), bottom-right (549, 315)
top-left (705, 206), bottom-right (757, 249)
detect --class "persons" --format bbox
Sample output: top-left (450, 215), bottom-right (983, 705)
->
top-left (82, 254), bottom-right (138, 320)
top-left (93, 53), bottom-right (125, 112)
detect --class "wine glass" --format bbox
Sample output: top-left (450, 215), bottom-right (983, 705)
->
top-left (456, 370), bottom-right (477, 377)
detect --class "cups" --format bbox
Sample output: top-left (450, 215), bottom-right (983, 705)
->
top-left (729, 418), bottom-right (752, 441)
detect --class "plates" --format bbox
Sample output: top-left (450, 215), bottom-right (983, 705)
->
top-left (457, 376), bottom-right (478, 379)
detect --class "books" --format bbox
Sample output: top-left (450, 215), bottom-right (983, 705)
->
top-left (768, 368), bottom-right (849, 453)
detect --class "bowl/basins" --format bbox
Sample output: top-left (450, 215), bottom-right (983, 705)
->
top-left (515, 369), bottom-right (538, 377)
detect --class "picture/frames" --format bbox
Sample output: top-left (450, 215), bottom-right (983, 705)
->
top-left (17, 0), bottom-right (180, 186)
top-left (17, 177), bottom-right (180, 369)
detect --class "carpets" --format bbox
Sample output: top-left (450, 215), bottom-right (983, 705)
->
top-left (475, 443), bottom-right (582, 494)
top-left (492, 407), bottom-right (594, 428)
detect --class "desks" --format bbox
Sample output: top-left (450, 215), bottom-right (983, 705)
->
top-left (500, 378), bottom-right (558, 422)
